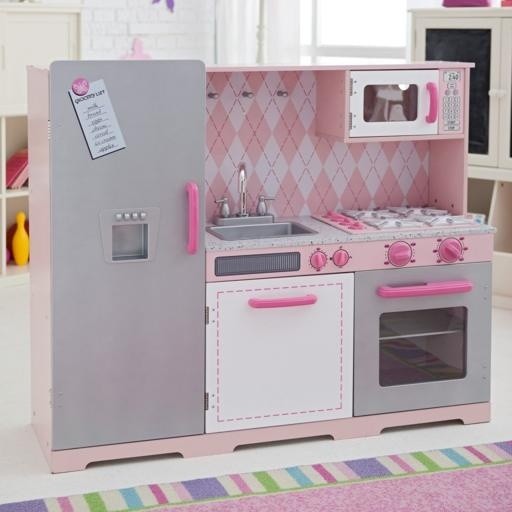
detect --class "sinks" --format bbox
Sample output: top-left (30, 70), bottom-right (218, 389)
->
top-left (206, 219), bottom-right (318, 241)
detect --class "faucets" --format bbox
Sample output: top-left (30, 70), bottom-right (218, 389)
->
top-left (236, 165), bottom-right (249, 217)
